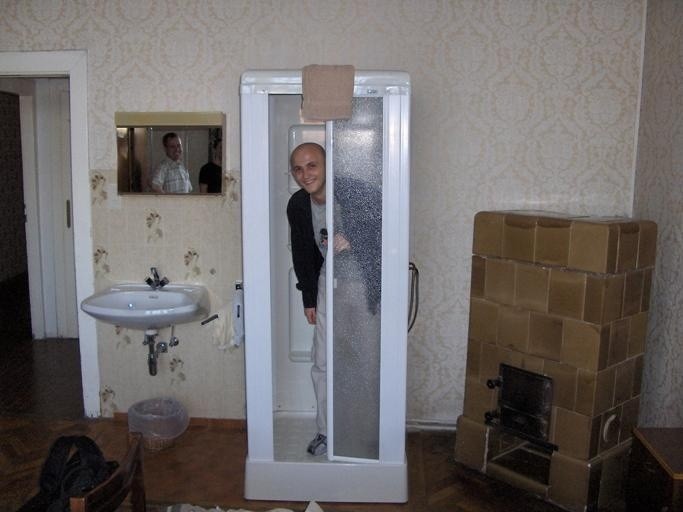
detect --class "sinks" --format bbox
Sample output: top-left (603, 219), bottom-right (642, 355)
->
top-left (80, 284), bottom-right (211, 331)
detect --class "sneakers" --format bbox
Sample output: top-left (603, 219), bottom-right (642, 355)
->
top-left (307, 434), bottom-right (333, 456)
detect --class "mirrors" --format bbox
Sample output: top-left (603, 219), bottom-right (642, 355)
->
top-left (114, 114), bottom-right (226, 196)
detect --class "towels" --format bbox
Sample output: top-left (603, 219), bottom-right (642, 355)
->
top-left (299, 63), bottom-right (354, 121)
top-left (213, 307), bottom-right (237, 357)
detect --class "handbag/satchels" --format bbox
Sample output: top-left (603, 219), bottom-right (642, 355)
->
top-left (14, 434), bottom-right (119, 512)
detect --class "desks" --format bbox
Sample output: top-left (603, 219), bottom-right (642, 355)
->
top-left (625, 421), bottom-right (682, 512)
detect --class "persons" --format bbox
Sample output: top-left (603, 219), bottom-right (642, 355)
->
top-left (286, 142), bottom-right (381, 456)
top-left (197, 138), bottom-right (221, 194)
top-left (146, 133), bottom-right (192, 194)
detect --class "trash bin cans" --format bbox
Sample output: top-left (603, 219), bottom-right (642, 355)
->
top-left (128, 397), bottom-right (183, 451)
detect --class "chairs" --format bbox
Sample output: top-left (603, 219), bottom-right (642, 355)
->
top-left (69, 427), bottom-right (148, 510)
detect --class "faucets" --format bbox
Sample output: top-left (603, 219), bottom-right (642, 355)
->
top-left (143, 267), bottom-right (169, 291)
